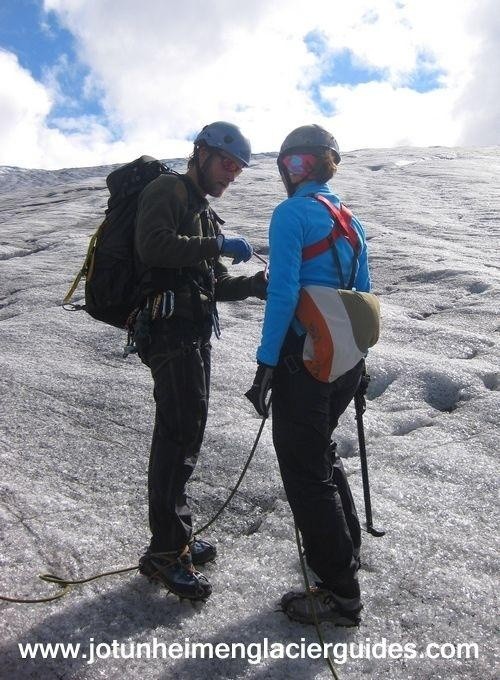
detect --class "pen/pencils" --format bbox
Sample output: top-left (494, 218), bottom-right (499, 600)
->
top-left (253, 251), bottom-right (268, 266)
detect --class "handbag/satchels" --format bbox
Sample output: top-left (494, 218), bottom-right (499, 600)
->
top-left (294, 285), bottom-right (380, 382)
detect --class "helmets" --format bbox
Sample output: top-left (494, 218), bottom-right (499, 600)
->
top-left (279, 125), bottom-right (340, 165)
top-left (194, 122), bottom-right (251, 167)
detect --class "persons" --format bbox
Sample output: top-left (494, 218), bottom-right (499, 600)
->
top-left (244, 124), bottom-right (369, 630)
top-left (130, 123), bottom-right (271, 602)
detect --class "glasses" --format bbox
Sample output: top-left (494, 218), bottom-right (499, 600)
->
top-left (216, 150), bottom-right (242, 178)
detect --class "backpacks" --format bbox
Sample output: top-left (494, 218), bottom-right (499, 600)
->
top-left (83, 155), bottom-right (218, 328)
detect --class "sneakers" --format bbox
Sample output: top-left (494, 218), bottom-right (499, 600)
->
top-left (280, 588), bottom-right (362, 627)
top-left (139, 540), bottom-right (211, 603)
top-left (189, 539), bottom-right (217, 565)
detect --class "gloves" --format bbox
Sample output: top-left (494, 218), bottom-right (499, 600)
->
top-left (216, 233), bottom-right (253, 265)
top-left (244, 366), bottom-right (273, 418)
top-left (232, 270), bottom-right (269, 300)
